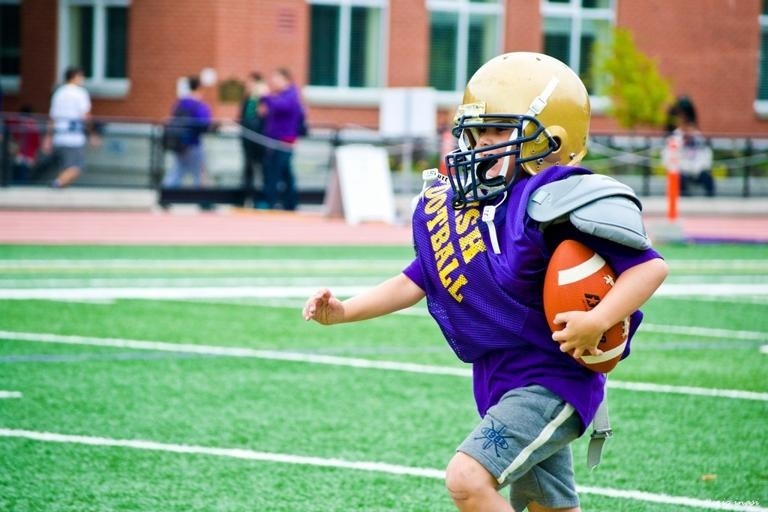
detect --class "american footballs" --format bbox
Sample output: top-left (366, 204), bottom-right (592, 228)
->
top-left (544, 240), bottom-right (631, 373)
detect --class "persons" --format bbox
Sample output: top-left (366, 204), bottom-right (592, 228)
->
top-left (8, 103), bottom-right (41, 178)
top-left (241, 67), bottom-right (303, 210)
top-left (158, 75), bottom-right (217, 209)
top-left (301, 52), bottom-right (668, 512)
top-left (660, 118), bottom-right (715, 196)
top-left (43, 69), bottom-right (102, 187)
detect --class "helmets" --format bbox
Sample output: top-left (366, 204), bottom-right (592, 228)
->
top-left (445, 52), bottom-right (590, 211)
